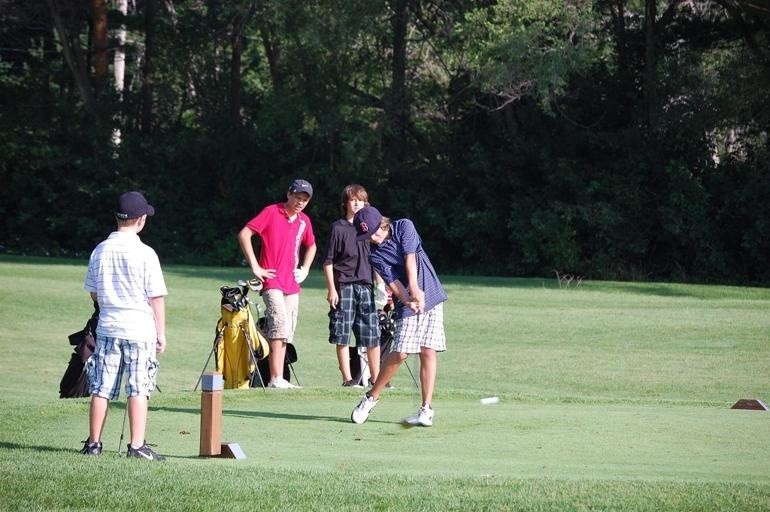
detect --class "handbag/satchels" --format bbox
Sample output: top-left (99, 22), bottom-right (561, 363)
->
top-left (59, 301), bottom-right (100, 398)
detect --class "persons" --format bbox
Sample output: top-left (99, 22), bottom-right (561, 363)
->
top-left (321, 183), bottom-right (396, 390)
top-left (236, 177), bottom-right (318, 393)
top-left (78, 190), bottom-right (168, 463)
top-left (350, 205), bottom-right (449, 428)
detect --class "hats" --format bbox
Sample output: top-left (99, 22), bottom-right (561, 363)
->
top-left (115, 191), bottom-right (155, 220)
top-left (353, 207), bottom-right (382, 241)
top-left (289, 179), bottom-right (313, 197)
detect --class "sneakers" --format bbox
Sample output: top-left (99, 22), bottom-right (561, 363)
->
top-left (268, 378), bottom-right (301, 388)
top-left (127, 440), bottom-right (167, 461)
top-left (418, 404), bottom-right (434, 426)
top-left (351, 392), bottom-right (379, 423)
top-left (79, 436), bottom-right (102, 455)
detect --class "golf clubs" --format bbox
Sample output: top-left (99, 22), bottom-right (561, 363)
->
top-left (192, 277), bottom-right (301, 393)
top-left (404, 311), bottom-right (423, 426)
